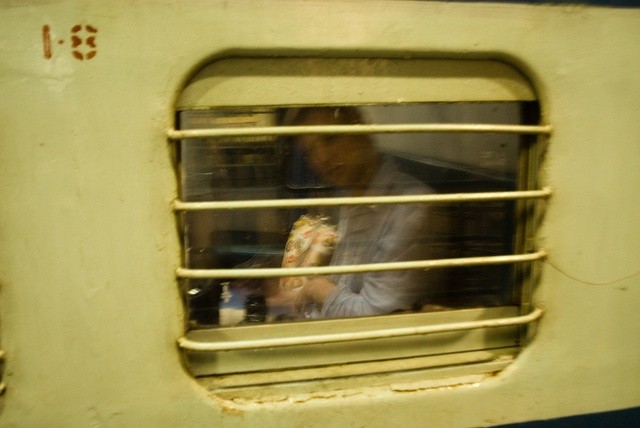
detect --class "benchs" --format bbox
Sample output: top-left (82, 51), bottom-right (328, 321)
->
top-left (386, 149), bottom-right (517, 304)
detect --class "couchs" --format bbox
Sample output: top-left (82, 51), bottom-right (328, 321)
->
top-left (208, 146), bottom-right (312, 268)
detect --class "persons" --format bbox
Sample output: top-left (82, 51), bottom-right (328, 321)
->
top-left (278, 214), bottom-right (288, 217)
top-left (264, 104), bottom-right (445, 323)
